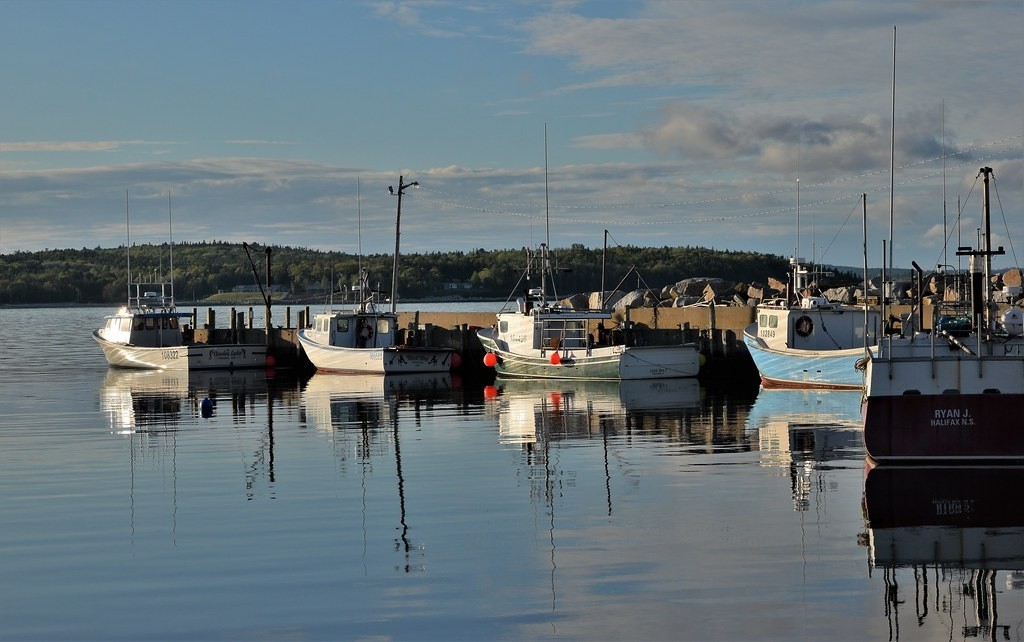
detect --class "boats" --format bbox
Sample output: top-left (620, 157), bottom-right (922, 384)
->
top-left (485, 375), bottom-right (706, 634)
top-left (475, 122), bottom-right (700, 381)
top-left (860, 24), bottom-right (1024, 463)
top-left (744, 376), bottom-right (866, 578)
top-left (295, 176), bottom-right (457, 373)
top-left (91, 190), bottom-right (268, 370)
top-left (742, 137), bottom-right (883, 390)
top-left (100, 366), bottom-right (268, 548)
top-left (301, 370), bottom-right (458, 573)
top-left (860, 455), bottom-right (1023, 641)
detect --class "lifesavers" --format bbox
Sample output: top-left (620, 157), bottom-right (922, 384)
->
top-left (795, 315), bottom-right (813, 337)
top-left (358, 325), bottom-right (373, 340)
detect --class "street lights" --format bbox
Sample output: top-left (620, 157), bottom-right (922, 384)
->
top-left (387, 175), bottom-right (420, 315)
